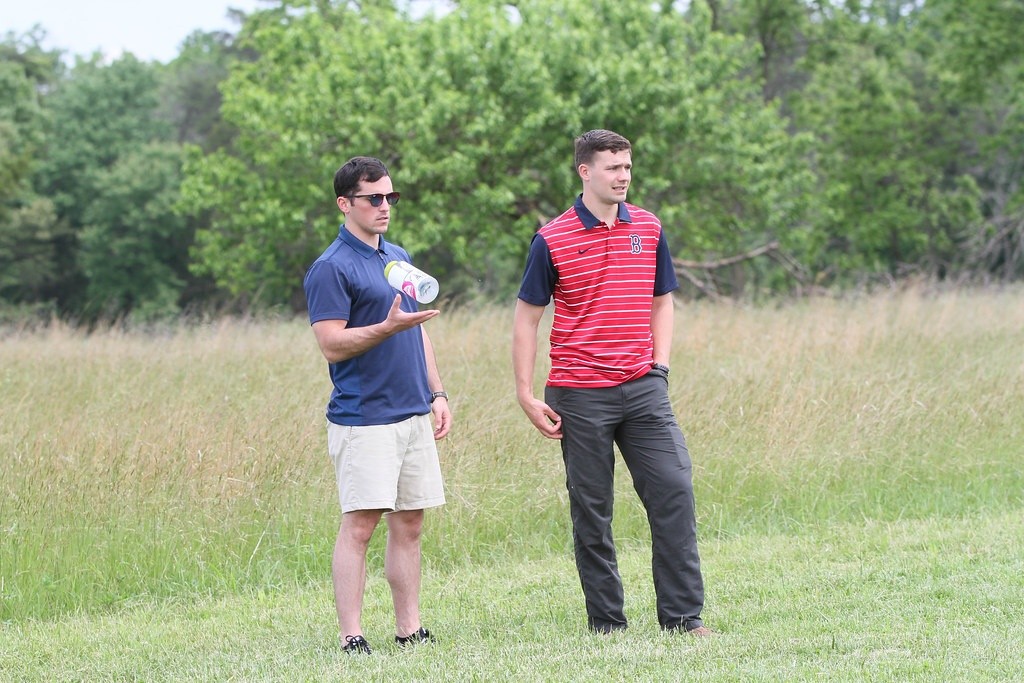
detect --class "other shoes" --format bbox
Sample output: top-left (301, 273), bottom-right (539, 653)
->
top-left (688, 627), bottom-right (720, 637)
top-left (340, 635), bottom-right (372, 655)
top-left (395, 627), bottom-right (443, 652)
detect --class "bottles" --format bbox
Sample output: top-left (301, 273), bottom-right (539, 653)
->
top-left (384, 260), bottom-right (440, 304)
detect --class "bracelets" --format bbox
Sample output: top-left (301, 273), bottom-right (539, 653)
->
top-left (651, 363), bottom-right (670, 374)
top-left (430, 391), bottom-right (449, 404)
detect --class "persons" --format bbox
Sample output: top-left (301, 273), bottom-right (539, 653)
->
top-left (509, 126), bottom-right (716, 644)
top-left (301, 155), bottom-right (453, 659)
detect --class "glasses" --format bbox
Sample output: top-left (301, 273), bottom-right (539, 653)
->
top-left (345, 192), bottom-right (401, 206)
